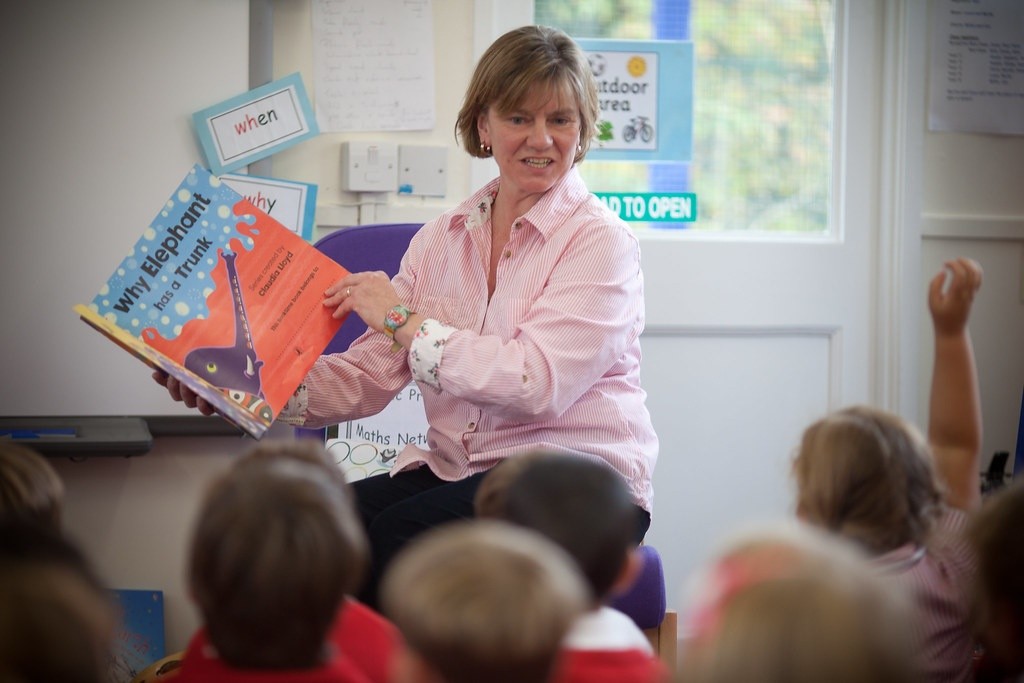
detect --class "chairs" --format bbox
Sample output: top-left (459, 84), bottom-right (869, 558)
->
top-left (292, 221), bottom-right (668, 628)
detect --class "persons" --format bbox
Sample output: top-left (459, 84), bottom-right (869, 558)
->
top-left (475, 447), bottom-right (669, 682)
top-left (680, 534), bottom-right (916, 683)
top-left (793, 257), bottom-right (987, 648)
top-left (958, 472), bottom-right (1024, 682)
top-left (178, 455), bottom-right (370, 683)
top-left (183, 438), bottom-right (440, 682)
top-left (153, 25), bottom-right (659, 617)
top-left (1, 442), bottom-right (126, 682)
top-left (381, 518), bottom-right (594, 682)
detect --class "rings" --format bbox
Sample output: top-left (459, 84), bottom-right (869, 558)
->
top-left (346, 285), bottom-right (351, 296)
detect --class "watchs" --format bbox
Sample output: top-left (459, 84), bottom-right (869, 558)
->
top-left (383, 304), bottom-right (412, 353)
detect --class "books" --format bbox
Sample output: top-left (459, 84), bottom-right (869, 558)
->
top-left (74, 162), bottom-right (354, 441)
